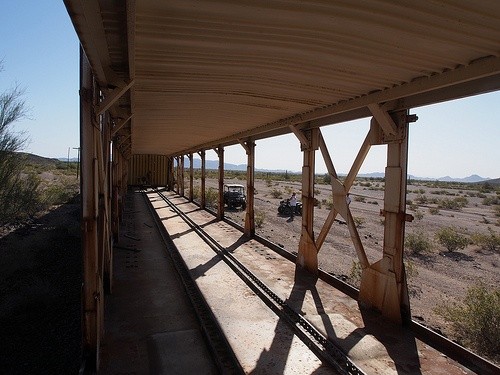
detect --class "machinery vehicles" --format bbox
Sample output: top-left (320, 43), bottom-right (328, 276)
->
top-left (224, 183), bottom-right (247, 210)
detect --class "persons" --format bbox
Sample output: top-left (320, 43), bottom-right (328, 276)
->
top-left (346, 193), bottom-right (351, 206)
top-left (289, 193), bottom-right (296, 217)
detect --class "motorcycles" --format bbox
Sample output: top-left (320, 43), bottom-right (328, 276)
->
top-left (278, 199), bottom-right (302, 216)
